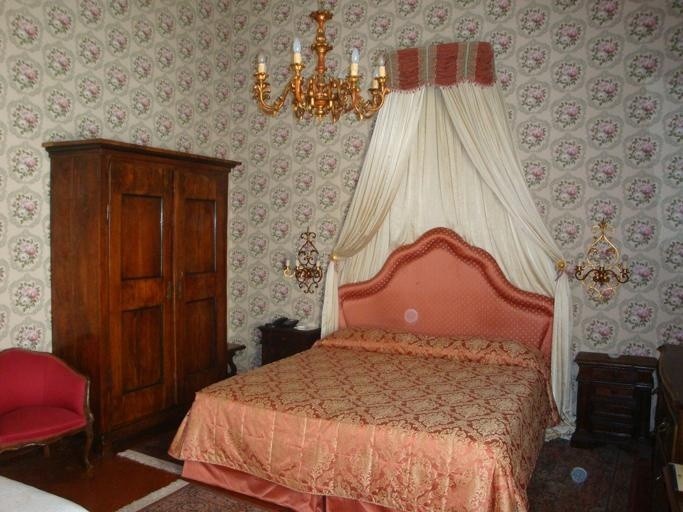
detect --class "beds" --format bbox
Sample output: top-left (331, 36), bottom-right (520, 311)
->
top-left (174, 225), bottom-right (563, 512)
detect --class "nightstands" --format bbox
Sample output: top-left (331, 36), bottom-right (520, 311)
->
top-left (567, 350), bottom-right (662, 458)
top-left (257, 317), bottom-right (321, 365)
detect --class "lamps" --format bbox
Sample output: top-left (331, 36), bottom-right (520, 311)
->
top-left (572, 215), bottom-right (631, 303)
top-left (250, 2), bottom-right (391, 126)
top-left (280, 224), bottom-right (328, 297)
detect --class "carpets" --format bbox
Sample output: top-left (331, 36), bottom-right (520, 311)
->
top-left (0, 434), bottom-right (641, 512)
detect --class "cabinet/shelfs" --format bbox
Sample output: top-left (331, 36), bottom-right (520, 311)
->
top-left (39, 138), bottom-right (243, 456)
top-left (647, 338), bottom-right (681, 512)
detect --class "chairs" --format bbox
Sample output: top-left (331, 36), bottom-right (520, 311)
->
top-left (0, 345), bottom-right (96, 481)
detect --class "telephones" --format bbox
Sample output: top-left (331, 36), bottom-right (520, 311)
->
top-left (273, 317), bottom-right (299, 328)
